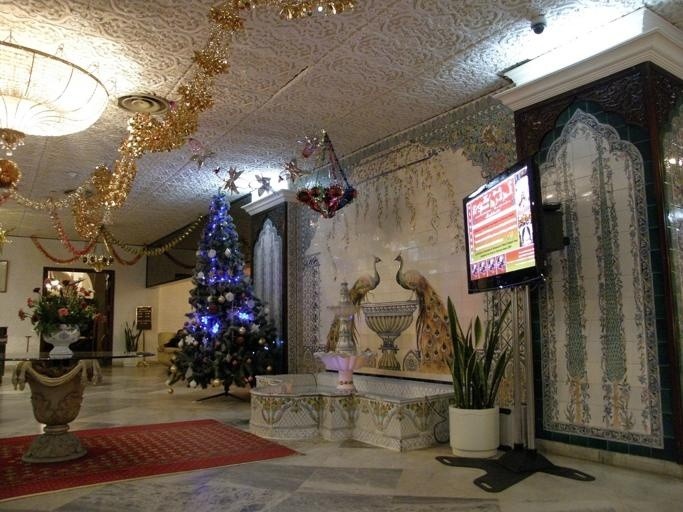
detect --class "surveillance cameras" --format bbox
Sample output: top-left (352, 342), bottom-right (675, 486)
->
top-left (531, 14), bottom-right (546, 35)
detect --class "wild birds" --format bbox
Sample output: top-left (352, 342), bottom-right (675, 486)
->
top-left (325, 254), bottom-right (384, 352)
top-left (393, 251), bottom-right (453, 374)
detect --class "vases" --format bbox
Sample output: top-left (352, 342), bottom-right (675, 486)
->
top-left (42, 323), bottom-right (81, 355)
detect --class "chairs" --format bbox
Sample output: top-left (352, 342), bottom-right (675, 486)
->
top-left (157, 332), bottom-right (179, 368)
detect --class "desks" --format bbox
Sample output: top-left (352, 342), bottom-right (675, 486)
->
top-left (0, 352), bottom-right (154, 462)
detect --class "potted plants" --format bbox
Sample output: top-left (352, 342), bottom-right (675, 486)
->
top-left (124, 320), bottom-right (143, 367)
top-left (444, 296), bottom-right (524, 458)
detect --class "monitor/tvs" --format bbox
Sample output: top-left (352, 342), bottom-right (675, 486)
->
top-left (462, 156), bottom-right (546, 293)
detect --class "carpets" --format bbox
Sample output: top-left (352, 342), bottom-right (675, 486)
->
top-left (1, 418), bottom-right (305, 500)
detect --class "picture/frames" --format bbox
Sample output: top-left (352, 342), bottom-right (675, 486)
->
top-left (1, 260), bottom-right (9, 293)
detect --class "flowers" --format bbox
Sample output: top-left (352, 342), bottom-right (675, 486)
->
top-left (18, 280), bottom-right (107, 337)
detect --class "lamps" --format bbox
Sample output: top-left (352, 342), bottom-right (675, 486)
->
top-left (0, 41), bottom-right (108, 156)
